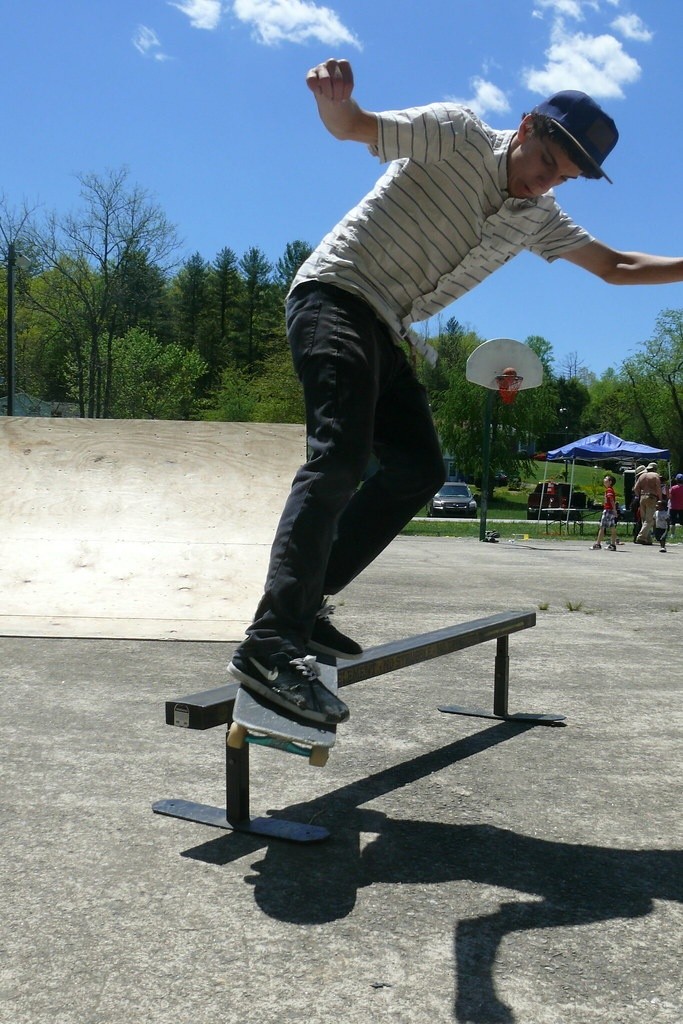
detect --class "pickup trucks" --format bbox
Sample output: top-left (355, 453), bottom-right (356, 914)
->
top-left (527, 481), bottom-right (575, 521)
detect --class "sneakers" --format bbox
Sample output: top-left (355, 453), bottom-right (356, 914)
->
top-left (226, 649), bottom-right (351, 724)
top-left (307, 613), bottom-right (363, 660)
top-left (605, 544), bottom-right (617, 551)
top-left (590, 544), bottom-right (601, 549)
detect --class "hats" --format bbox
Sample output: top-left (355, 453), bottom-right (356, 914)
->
top-left (647, 463), bottom-right (658, 470)
top-left (534, 90), bottom-right (618, 183)
top-left (634, 465), bottom-right (647, 477)
top-left (675, 473), bottom-right (683, 480)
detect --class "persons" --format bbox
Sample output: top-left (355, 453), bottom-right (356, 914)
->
top-left (225, 54), bottom-right (683, 726)
top-left (669, 474), bottom-right (683, 525)
top-left (631, 462), bottom-right (663, 545)
top-left (589, 474), bottom-right (619, 552)
top-left (652, 500), bottom-right (671, 552)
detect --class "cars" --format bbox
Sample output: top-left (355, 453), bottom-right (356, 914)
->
top-left (426, 482), bottom-right (478, 519)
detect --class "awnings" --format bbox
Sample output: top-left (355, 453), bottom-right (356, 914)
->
top-left (537, 431), bottom-right (671, 538)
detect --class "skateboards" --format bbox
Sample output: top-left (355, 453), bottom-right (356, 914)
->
top-left (228, 589), bottom-right (338, 767)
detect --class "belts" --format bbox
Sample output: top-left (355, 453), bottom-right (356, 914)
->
top-left (640, 493), bottom-right (658, 497)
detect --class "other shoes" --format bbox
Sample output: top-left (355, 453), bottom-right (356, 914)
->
top-left (636, 539), bottom-right (652, 545)
top-left (660, 548), bottom-right (667, 552)
